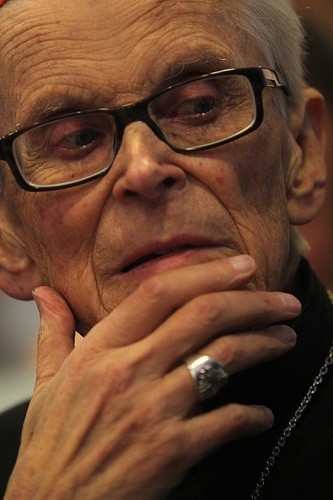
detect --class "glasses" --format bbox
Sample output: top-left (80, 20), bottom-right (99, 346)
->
top-left (0, 65), bottom-right (285, 193)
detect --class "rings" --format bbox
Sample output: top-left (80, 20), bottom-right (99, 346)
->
top-left (184, 354), bottom-right (229, 399)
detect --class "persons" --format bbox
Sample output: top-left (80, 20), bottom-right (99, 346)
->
top-left (0, 0), bottom-right (333, 500)
